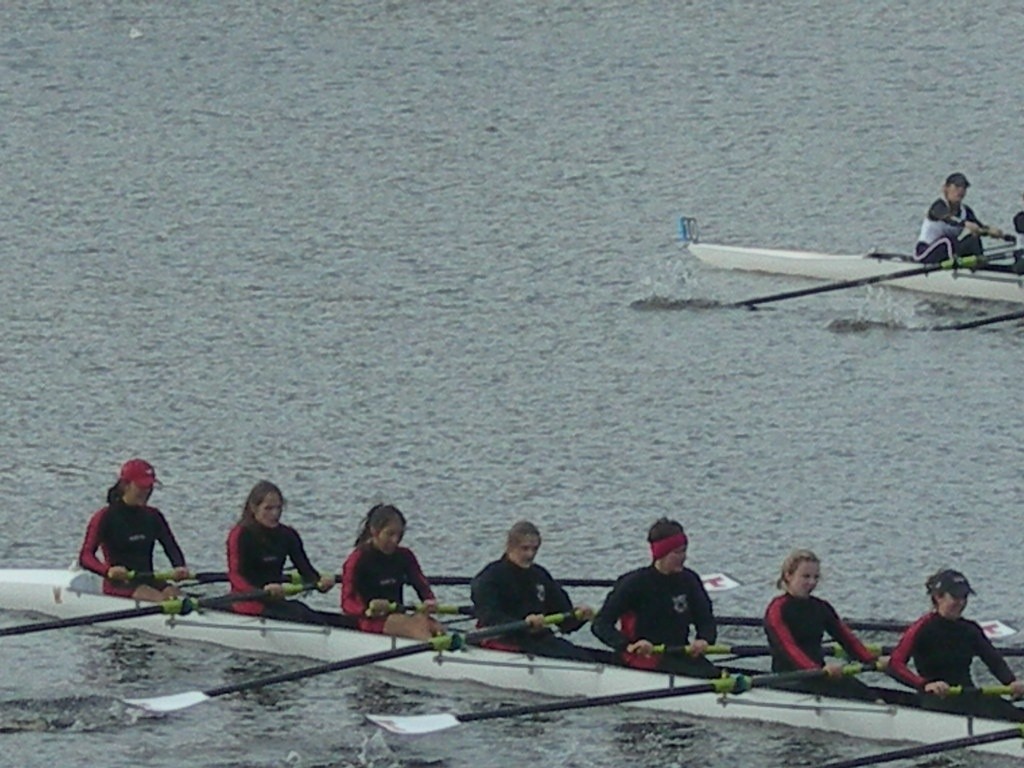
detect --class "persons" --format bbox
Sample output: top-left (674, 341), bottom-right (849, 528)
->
top-left (913, 171), bottom-right (1002, 270)
top-left (340, 503), bottom-right (446, 642)
top-left (79, 459), bottom-right (187, 602)
top-left (590, 517), bottom-right (722, 680)
top-left (762, 550), bottom-right (891, 690)
top-left (469, 518), bottom-right (592, 659)
top-left (222, 479), bottom-right (336, 626)
top-left (884, 570), bottom-right (1024, 703)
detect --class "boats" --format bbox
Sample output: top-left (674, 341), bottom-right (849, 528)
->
top-left (1, 567), bottom-right (1024, 762)
top-left (679, 213), bottom-right (1024, 307)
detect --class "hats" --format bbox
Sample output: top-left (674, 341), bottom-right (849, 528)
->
top-left (946, 173), bottom-right (970, 188)
top-left (926, 570), bottom-right (977, 598)
top-left (120, 458), bottom-right (163, 489)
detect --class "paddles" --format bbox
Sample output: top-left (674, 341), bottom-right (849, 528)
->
top-left (123, 610), bottom-right (568, 710)
top-left (365, 663), bottom-right (878, 738)
top-left (586, 610), bottom-right (1018, 642)
top-left (128, 570), bottom-right (301, 583)
top-left (820, 726), bottom-right (1023, 768)
top-left (644, 643), bottom-right (1024, 664)
top-left (931, 679), bottom-right (1014, 694)
top-left (2, 589), bottom-right (316, 638)
top-left (331, 571), bottom-right (743, 595)
top-left (730, 244), bottom-right (1024, 307)
